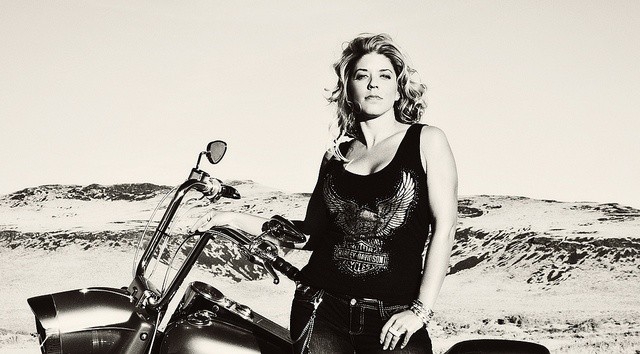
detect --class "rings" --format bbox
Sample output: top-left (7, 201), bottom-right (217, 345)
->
top-left (388, 327), bottom-right (401, 339)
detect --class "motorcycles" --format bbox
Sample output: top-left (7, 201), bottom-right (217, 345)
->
top-left (27, 139), bottom-right (550, 354)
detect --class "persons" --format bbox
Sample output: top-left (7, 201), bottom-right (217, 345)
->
top-left (189, 31), bottom-right (459, 353)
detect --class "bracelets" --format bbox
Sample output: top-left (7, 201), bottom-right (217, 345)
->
top-left (408, 299), bottom-right (434, 324)
